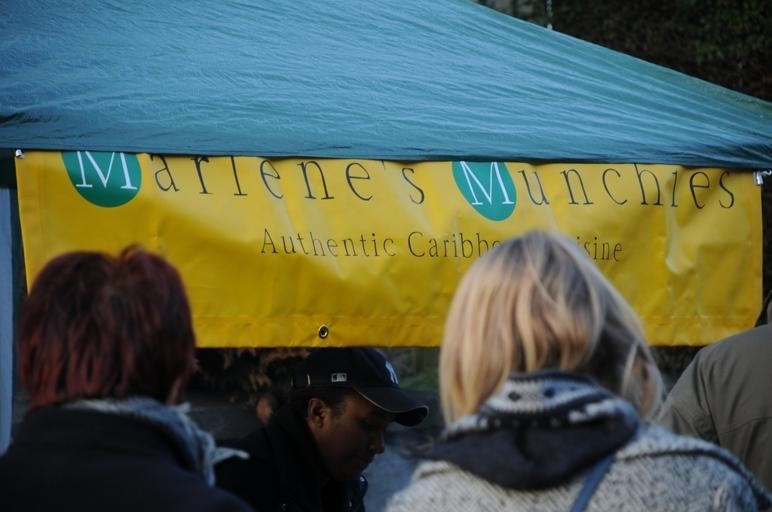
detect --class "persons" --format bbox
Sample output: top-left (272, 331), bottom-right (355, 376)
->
top-left (650, 291), bottom-right (772, 499)
top-left (386, 228), bottom-right (772, 512)
top-left (1, 241), bottom-right (254, 512)
top-left (175, 346), bottom-right (429, 511)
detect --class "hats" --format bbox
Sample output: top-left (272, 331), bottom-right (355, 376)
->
top-left (286, 347), bottom-right (429, 426)
